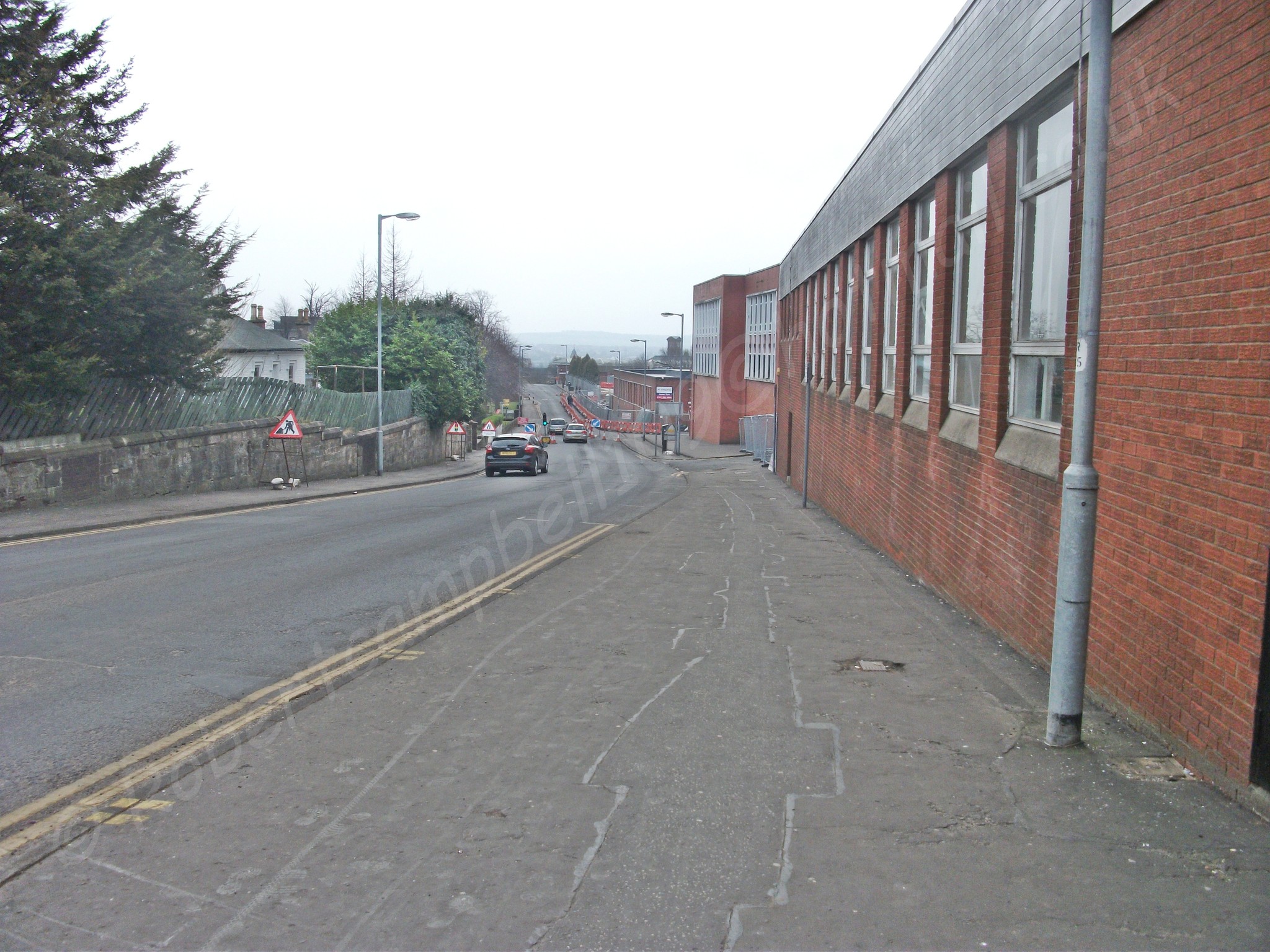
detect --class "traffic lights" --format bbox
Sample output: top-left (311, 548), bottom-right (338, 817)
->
top-left (542, 414), bottom-right (547, 425)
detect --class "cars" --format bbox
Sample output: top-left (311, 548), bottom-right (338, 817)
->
top-left (484, 433), bottom-right (549, 477)
top-left (546, 418), bottom-right (568, 435)
top-left (562, 424), bottom-right (587, 444)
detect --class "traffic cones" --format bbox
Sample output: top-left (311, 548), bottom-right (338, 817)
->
top-left (601, 431), bottom-right (606, 440)
top-left (550, 432), bottom-right (557, 444)
top-left (588, 426), bottom-right (595, 439)
top-left (617, 432), bottom-right (620, 442)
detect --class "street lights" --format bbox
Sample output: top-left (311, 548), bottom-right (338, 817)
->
top-left (560, 345), bottom-right (567, 385)
top-left (518, 345), bottom-right (532, 417)
top-left (660, 312), bottom-right (684, 455)
top-left (630, 339), bottom-right (647, 441)
top-left (609, 350), bottom-right (620, 398)
top-left (377, 210), bottom-right (421, 474)
